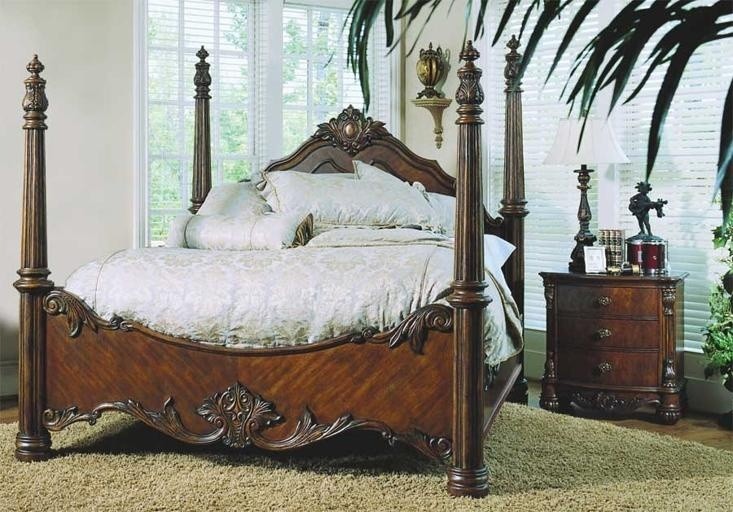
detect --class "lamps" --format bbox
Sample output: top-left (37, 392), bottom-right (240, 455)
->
top-left (413, 40), bottom-right (448, 98)
top-left (542, 114), bottom-right (632, 272)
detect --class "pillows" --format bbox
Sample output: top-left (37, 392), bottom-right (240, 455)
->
top-left (162, 159), bottom-right (457, 252)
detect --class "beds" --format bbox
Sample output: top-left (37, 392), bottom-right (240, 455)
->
top-left (13, 35), bottom-right (530, 502)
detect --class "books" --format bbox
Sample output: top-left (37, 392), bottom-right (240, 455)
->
top-left (599, 230), bottom-right (624, 267)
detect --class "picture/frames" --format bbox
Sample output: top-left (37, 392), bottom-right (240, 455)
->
top-left (581, 246), bottom-right (608, 276)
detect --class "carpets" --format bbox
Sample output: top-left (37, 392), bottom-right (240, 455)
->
top-left (0, 394), bottom-right (731, 511)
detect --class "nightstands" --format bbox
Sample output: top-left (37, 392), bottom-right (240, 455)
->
top-left (537, 266), bottom-right (689, 428)
